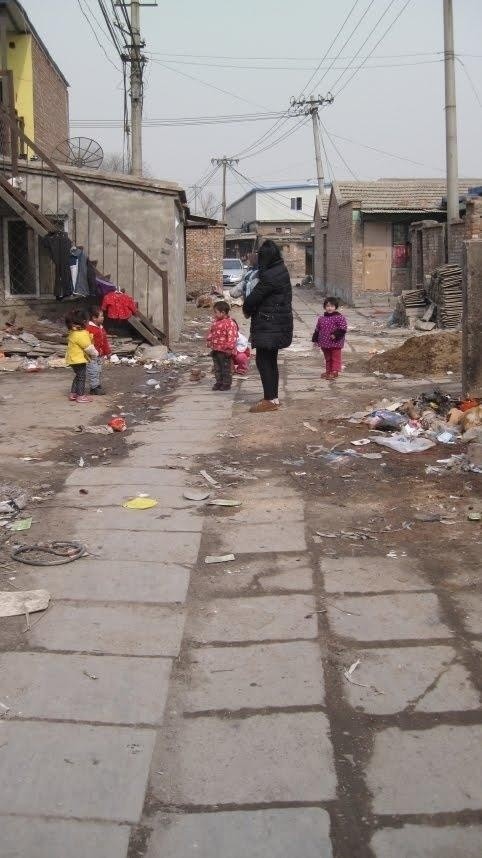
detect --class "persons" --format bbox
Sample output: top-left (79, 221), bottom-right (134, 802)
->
top-left (86, 306), bottom-right (112, 395)
top-left (207, 302), bottom-right (238, 391)
top-left (230, 319), bottom-right (250, 374)
top-left (242, 240), bottom-right (295, 413)
top-left (65, 308), bottom-right (98, 404)
top-left (312, 297), bottom-right (347, 380)
top-left (230, 254), bottom-right (261, 316)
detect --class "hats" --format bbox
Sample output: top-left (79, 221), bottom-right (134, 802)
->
top-left (223, 258), bottom-right (248, 286)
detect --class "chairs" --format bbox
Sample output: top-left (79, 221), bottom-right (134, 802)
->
top-left (249, 400), bottom-right (275, 412)
top-left (219, 384), bottom-right (230, 391)
top-left (321, 372), bottom-right (329, 378)
top-left (90, 387), bottom-right (106, 395)
top-left (69, 392), bottom-right (78, 401)
top-left (237, 370), bottom-right (247, 377)
top-left (212, 381), bottom-right (223, 390)
top-left (326, 371), bottom-right (338, 380)
top-left (77, 394), bottom-right (93, 402)
